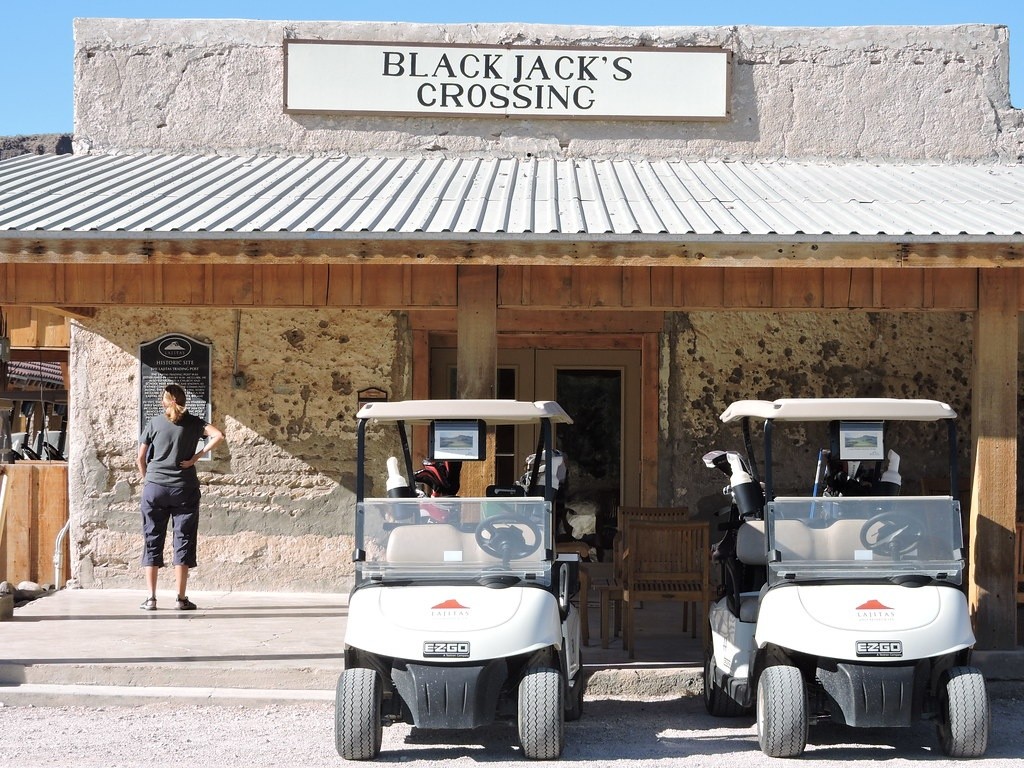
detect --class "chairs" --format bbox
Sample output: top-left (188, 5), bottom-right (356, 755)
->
top-left (556, 506), bottom-right (713, 658)
top-left (919, 477), bottom-right (972, 564)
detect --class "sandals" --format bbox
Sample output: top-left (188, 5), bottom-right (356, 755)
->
top-left (140, 597), bottom-right (156, 610)
top-left (176, 594), bottom-right (197, 610)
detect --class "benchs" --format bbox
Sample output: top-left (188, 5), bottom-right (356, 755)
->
top-left (739, 518), bottom-right (917, 623)
top-left (386, 524), bottom-right (548, 575)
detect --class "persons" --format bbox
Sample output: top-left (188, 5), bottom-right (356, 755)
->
top-left (136, 385), bottom-right (226, 610)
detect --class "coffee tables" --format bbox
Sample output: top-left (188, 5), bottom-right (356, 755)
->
top-left (590, 577), bottom-right (725, 650)
